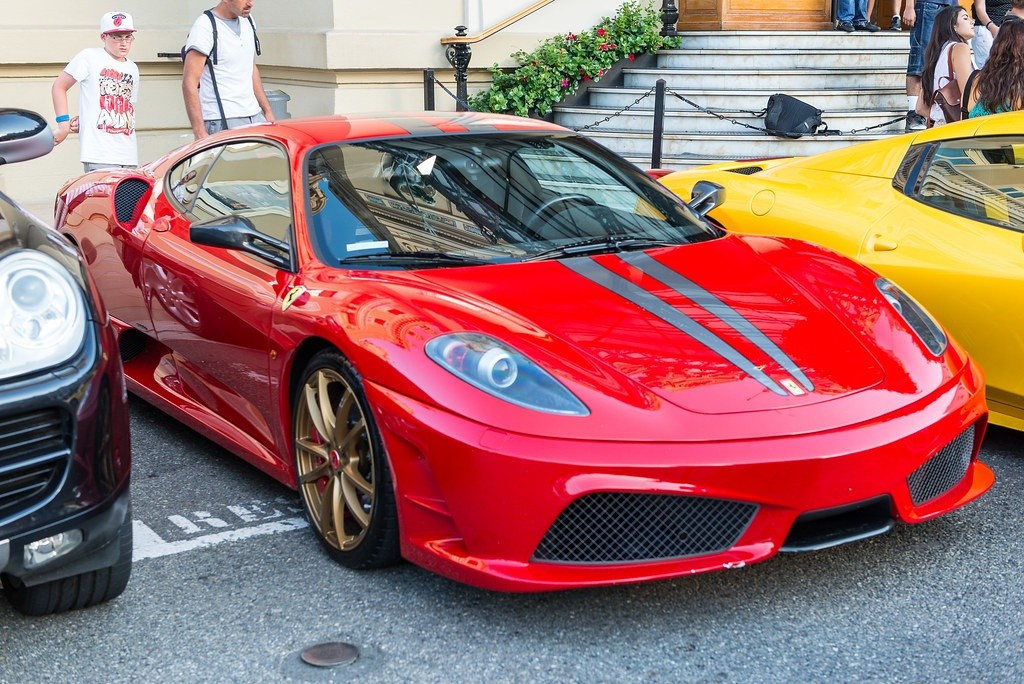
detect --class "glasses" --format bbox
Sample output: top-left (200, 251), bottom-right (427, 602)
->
top-left (108, 35), bottom-right (135, 43)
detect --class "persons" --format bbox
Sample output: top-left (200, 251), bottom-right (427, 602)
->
top-left (831, 0), bottom-right (1024, 134)
top-left (182, 0), bottom-right (275, 141)
top-left (54, 11), bottom-right (139, 172)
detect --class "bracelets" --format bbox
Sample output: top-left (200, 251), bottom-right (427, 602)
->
top-left (986, 21), bottom-right (992, 29)
top-left (56, 115), bottom-right (70, 123)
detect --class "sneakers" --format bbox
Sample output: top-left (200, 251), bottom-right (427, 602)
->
top-left (836, 22), bottom-right (855, 32)
top-left (854, 21), bottom-right (882, 32)
top-left (889, 15), bottom-right (902, 31)
top-left (906, 110), bottom-right (926, 131)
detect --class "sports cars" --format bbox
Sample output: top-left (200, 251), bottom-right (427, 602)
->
top-left (635, 109), bottom-right (1024, 432)
top-left (55, 109), bottom-right (997, 594)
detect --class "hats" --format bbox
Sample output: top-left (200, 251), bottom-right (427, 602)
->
top-left (100, 12), bottom-right (138, 35)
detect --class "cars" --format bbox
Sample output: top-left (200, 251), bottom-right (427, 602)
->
top-left (0, 107), bottom-right (135, 621)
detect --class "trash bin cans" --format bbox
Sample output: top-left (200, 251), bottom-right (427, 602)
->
top-left (265, 90), bottom-right (291, 120)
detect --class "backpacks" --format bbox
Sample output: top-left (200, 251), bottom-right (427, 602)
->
top-left (765, 94), bottom-right (823, 140)
top-left (934, 43), bottom-right (961, 123)
